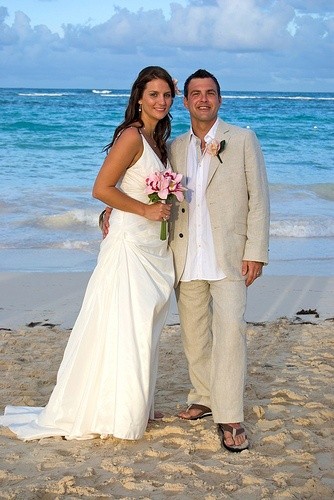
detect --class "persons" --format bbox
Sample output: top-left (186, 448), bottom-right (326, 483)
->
top-left (99, 68), bottom-right (271, 452)
top-left (0, 67), bottom-right (182, 442)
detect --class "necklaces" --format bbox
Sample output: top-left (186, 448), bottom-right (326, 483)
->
top-left (142, 128), bottom-right (157, 148)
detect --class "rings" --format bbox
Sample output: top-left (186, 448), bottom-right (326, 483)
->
top-left (257, 273), bottom-right (260, 275)
top-left (104, 210), bottom-right (110, 214)
top-left (164, 215), bottom-right (169, 219)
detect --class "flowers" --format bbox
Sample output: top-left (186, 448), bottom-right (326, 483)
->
top-left (205, 140), bottom-right (226, 164)
top-left (172, 78), bottom-right (183, 96)
top-left (145, 168), bottom-right (188, 241)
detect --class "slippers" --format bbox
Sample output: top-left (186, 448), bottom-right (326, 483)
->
top-left (177, 404), bottom-right (212, 420)
top-left (220, 424), bottom-right (249, 453)
